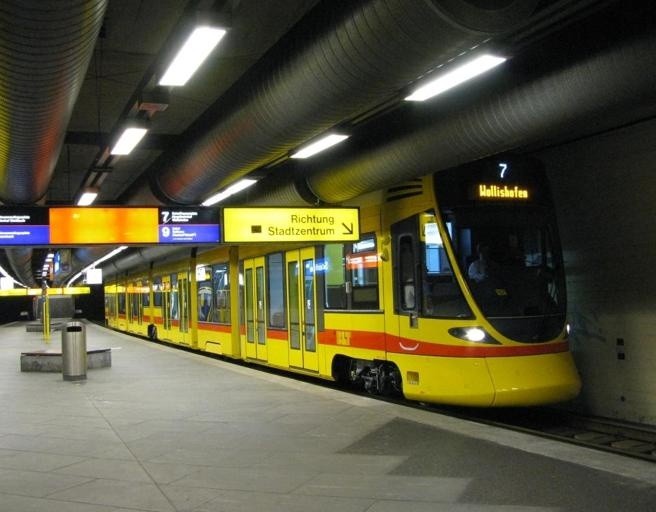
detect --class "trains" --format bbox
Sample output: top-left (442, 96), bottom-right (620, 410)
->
top-left (103, 155), bottom-right (583, 408)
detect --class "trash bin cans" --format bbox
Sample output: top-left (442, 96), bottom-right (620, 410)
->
top-left (61, 320), bottom-right (88, 381)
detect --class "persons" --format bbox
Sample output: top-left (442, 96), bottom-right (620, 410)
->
top-left (202, 300), bottom-right (210, 317)
top-left (468, 242), bottom-right (518, 286)
